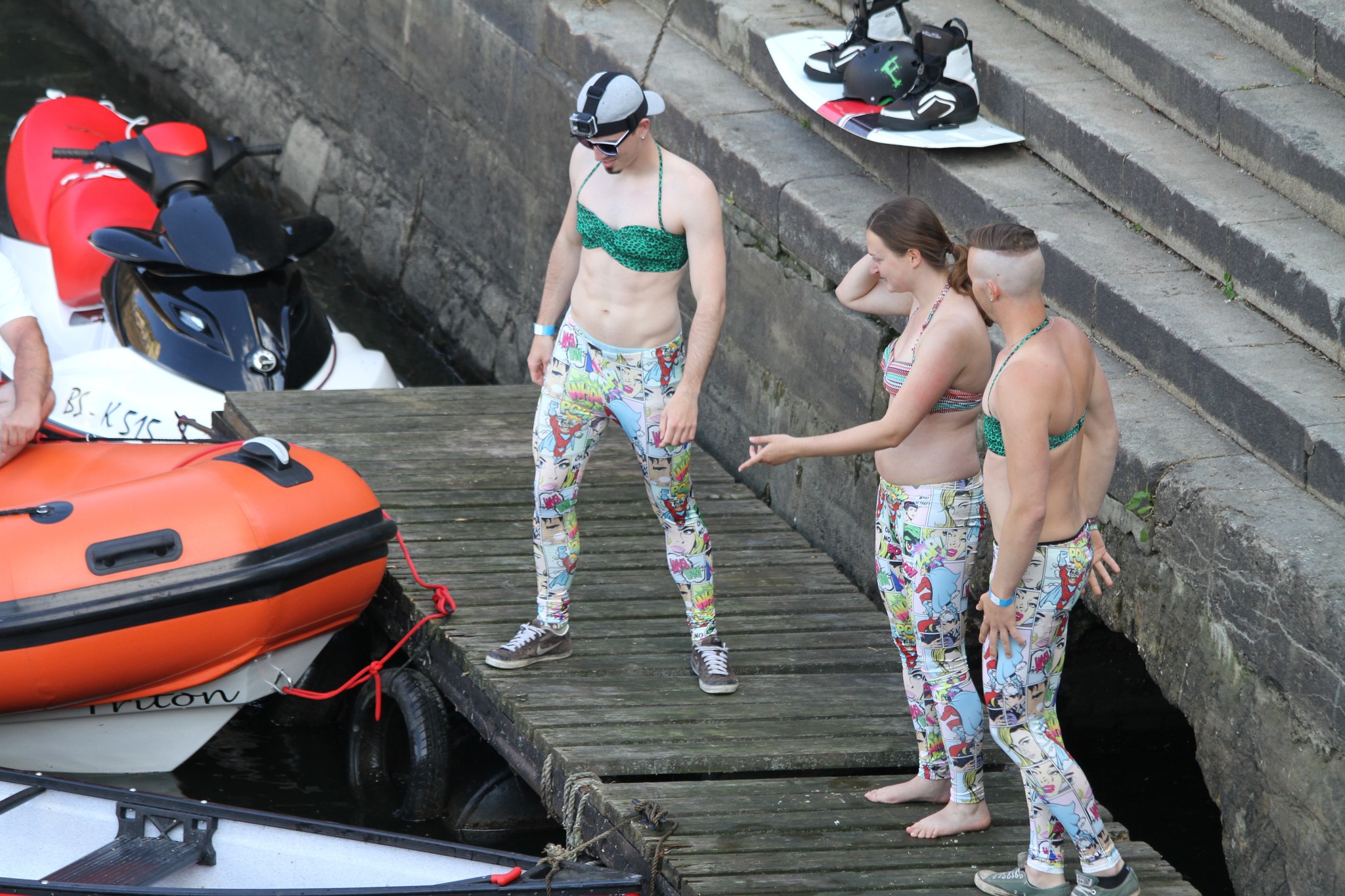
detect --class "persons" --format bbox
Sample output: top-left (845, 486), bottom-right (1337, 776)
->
top-left (962, 223), bottom-right (1141, 896)
top-left (1, 248), bottom-right (56, 475)
top-left (484, 70), bottom-right (739, 695)
top-left (737, 198), bottom-right (992, 839)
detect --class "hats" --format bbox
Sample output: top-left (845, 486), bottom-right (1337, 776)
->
top-left (569, 71), bottom-right (666, 130)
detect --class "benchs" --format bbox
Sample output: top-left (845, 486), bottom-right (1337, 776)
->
top-left (39, 835), bottom-right (201, 887)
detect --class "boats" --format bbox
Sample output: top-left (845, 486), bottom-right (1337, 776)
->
top-left (0, 765), bottom-right (648, 896)
top-left (0, 437), bottom-right (399, 778)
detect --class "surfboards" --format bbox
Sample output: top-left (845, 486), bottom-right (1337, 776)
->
top-left (764, 29), bottom-right (1027, 149)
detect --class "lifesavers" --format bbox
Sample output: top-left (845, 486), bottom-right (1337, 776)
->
top-left (344, 666), bottom-right (451, 838)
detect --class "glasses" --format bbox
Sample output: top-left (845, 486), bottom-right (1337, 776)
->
top-left (572, 129), bottom-right (633, 154)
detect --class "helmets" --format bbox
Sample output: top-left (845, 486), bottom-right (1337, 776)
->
top-left (841, 40), bottom-right (920, 104)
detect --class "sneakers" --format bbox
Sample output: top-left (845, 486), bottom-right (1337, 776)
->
top-left (485, 617), bottom-right (572, 669)
top-left (1070, 865), bottom-right (1143, 896)
top-left (877, 19), bottom-right (981, 130)
top-left (974, 863), bottom-right (1071, 896)
top-left (690, 636), bottom-right (739, 694)
top-left (803, 0), bottom-right (915, 83)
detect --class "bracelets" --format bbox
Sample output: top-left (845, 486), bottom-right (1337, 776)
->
top-left (988, 585), bottom-right (1015, 607)
top-left (1088, 516), bottom-right (1098, 530)
top-left (531, 323), bottom-right (556, 336)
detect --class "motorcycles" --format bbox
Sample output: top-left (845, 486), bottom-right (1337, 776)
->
top-left (0, 83), bottom-right (403, 449)
